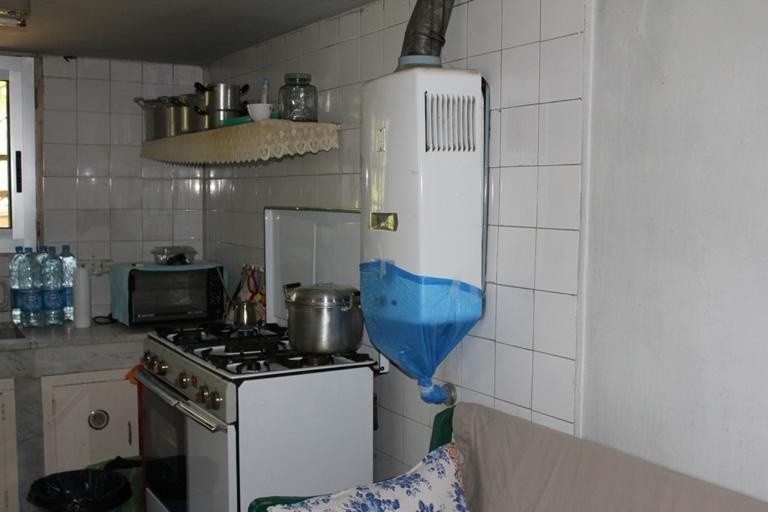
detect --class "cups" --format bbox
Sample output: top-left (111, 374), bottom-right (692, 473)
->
top-left (233, 301), bottom-right (256, 330)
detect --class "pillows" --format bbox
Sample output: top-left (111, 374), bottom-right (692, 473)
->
top-left (266, 440), bottom-right (470, 512)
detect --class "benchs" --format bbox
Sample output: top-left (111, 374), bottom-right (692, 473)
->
top-left (247, 400), bottom-right (768, 512)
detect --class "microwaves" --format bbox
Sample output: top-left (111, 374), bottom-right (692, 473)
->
top-left (110, 260), bottom-right (224, 330)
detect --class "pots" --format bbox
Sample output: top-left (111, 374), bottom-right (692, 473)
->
top-left (133, 81), bottom-right (249, 141)
top-left (150, 245), bottom-right (197, 266)
top-left (282, 281), bottom-right (364, 356)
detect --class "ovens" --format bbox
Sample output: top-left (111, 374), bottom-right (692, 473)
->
top-left (134, 368), bottom-right (189, 511)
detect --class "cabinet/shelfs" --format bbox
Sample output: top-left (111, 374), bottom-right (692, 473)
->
top-left (36, 367), bottom-right (140, 476)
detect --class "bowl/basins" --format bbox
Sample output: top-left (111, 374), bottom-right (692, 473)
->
top-left (246, 103), bottom-right (274, 122)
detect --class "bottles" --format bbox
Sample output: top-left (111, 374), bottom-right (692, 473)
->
top-left (8, 244), bottom-right (78, 330)
top-left (258, 78), bottom-right (270, 104)
top-left (277, 72), bottom-right (318, 123)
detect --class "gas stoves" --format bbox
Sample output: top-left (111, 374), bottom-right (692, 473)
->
top-left (141, 321), bottom-right (377, 425)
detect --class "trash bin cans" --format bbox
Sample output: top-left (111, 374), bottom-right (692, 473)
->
top-left (26, 468), bottom-right (132, 512)
